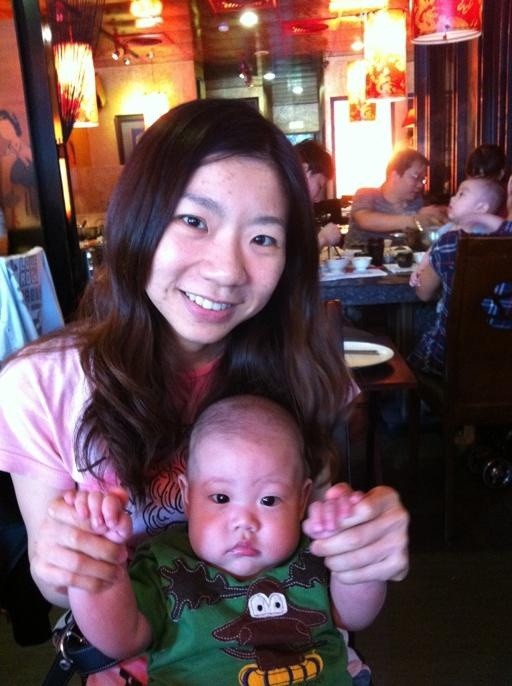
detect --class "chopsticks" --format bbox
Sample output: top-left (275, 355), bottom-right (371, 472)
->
top-left (326, 246), bottom-right (342, 260)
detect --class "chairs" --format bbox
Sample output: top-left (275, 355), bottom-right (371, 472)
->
top-left (406, 234), bottom-right (512, 546)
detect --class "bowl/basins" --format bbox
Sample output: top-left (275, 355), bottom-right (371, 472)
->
top-left (351, 256), bottom-right (374, 272)
top-left (325, 257), bottom-right (350, 274)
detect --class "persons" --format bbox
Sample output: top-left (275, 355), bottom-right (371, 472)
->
top-left (406, 175), bottom-right (507, 353)
top-left (0, 92), bottom-right (413, 686)
top-left (463, 143), bottom-right (511, 220)
top-left (406, 171), bottom-right (512, 475)
top-left (342, 149), bottom-right (449, 252)
top-left (291, 138), bottom-right (344, 257)
top-left (58, 387), bottom-right (391, 686)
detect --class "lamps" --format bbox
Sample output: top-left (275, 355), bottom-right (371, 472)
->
top-left (347, 0), bottom-right (481, 123)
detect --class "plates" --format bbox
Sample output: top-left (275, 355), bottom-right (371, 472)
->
top-left (344, 340), bottom-right (396, 370)
top-left (382, 262), bottom-right (419, 276)
